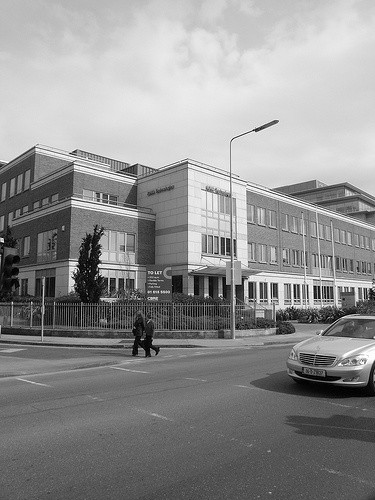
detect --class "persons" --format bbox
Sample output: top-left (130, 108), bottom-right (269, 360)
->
top-left (131, 310), bottom-right (146, 356)
top-left (144, 314), bottom-right (160, 358)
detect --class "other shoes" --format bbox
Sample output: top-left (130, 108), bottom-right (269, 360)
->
top-left (148, 354), bottom-right (151, 357)
top-left (132, 353), bottom-right (138, 356)
top-left (155, 350), bottom-right (160, 355)
top-left (145, 354), bottom-right (149, 357)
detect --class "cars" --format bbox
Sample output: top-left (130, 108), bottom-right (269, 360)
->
top-left (286, 313), bottom-right (375, 391)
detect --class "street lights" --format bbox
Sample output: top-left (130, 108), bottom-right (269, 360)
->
top-left (229, 119), bottom-right (279, 340)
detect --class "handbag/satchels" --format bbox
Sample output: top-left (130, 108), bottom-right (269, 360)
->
top-left (132, 320), bottom-right (138, 336)
